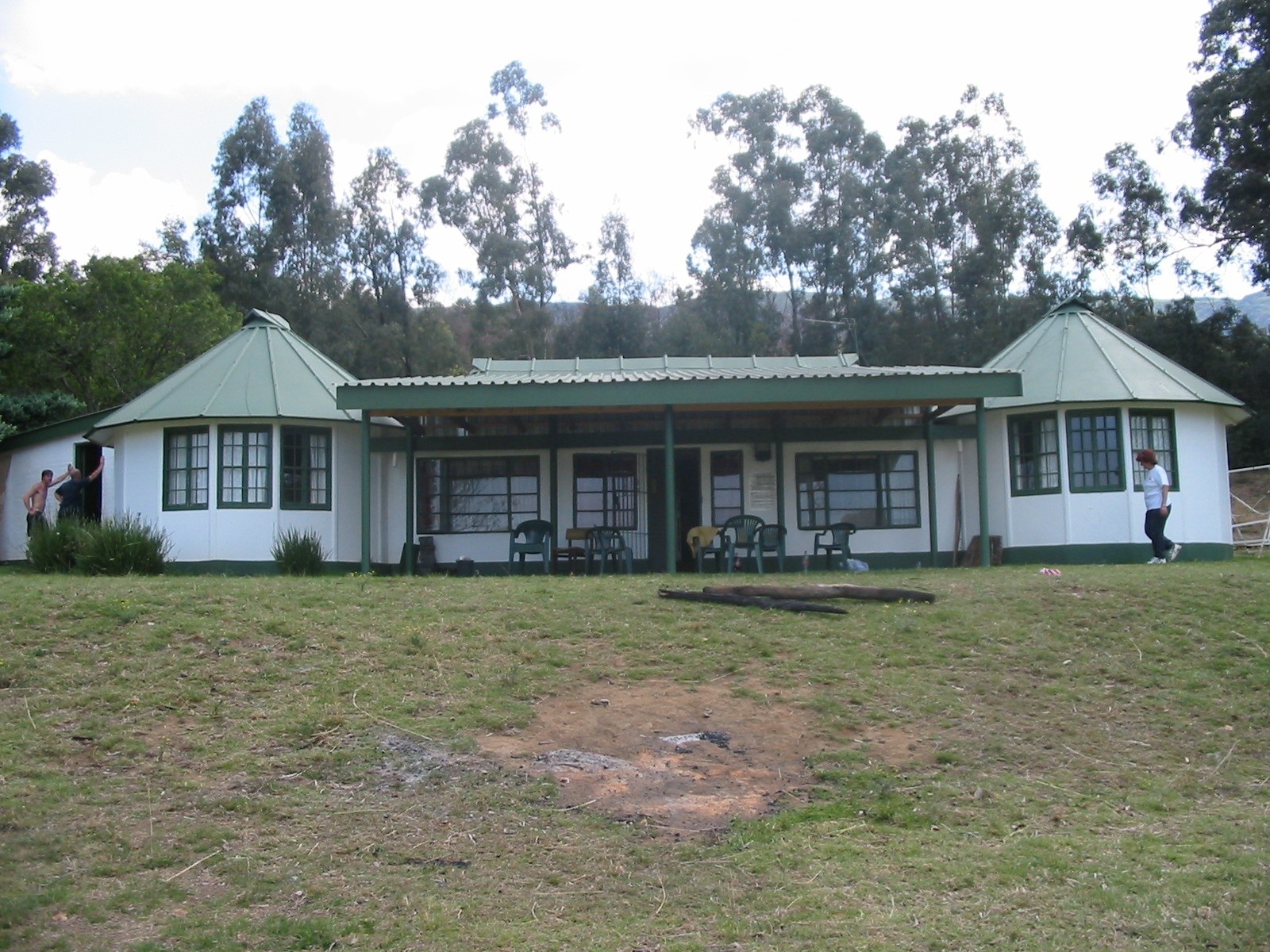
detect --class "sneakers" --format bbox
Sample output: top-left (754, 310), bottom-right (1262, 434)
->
top-left (1169, 544), bottom-right (1181, 561)
top-left (1148, 556), bottom-right (1167, 564)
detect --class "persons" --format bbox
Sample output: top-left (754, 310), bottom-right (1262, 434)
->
top-left (54, 456), bottom-right (105, 522)
top-left (1135, 449), bottom-right (1182, 565)
top-left (22, 463), bottom-right (72, 537)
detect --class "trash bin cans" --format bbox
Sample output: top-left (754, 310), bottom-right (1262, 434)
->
top-left (456, 560), bottom-right (474, 577)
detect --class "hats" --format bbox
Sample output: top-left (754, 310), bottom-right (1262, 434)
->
top-left (1136, 451), bottom-right (1158, 464)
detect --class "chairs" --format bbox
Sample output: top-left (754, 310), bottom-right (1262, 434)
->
top-left (688, 515), bottom-right (787, 575)
top-left (507, 520), bottom-right (634, 577)
top-left (813, 523), bottom-right (856, 571)
